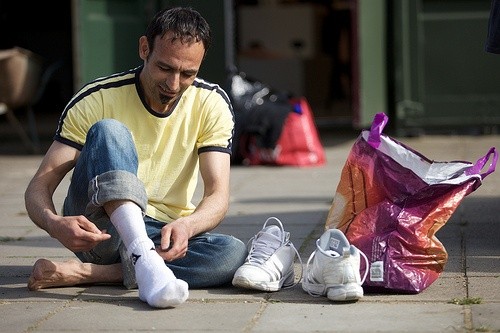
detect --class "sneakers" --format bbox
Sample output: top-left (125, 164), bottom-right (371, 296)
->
top-left (302, 229), bottom-right (369, 300)
top-left (232, 217), bottom-right (303, 291)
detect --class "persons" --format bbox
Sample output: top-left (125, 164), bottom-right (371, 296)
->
top-left (24, 5), bottom-right (250, 308)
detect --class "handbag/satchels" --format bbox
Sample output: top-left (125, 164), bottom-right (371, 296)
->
top-left (230, 86), bottom-right (327, 168)
top-left (323, 113), bottom-right (498, 293)
